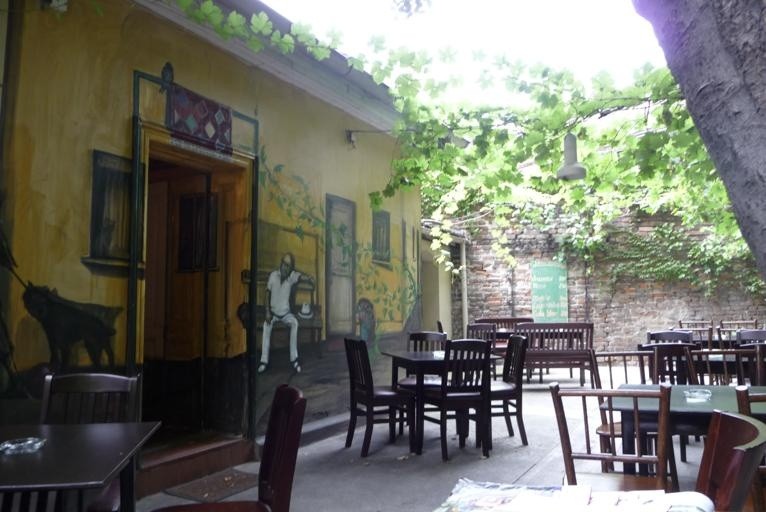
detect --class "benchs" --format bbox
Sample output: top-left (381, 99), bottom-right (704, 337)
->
top-left (513, 322), bottom-right (597, 389)
top-left (474, 317), bottom-right (534, 328)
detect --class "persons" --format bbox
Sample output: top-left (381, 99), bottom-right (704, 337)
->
top-left (257, 254), bottom-right (317, 373)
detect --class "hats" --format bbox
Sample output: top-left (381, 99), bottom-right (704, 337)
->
top-left (298, 302), bottom-right (314, 319)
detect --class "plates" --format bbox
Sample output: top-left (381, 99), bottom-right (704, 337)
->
top-left (297, 309), bottom-right (314, 320)
top-left (683, 390), bottom-right (712, 400)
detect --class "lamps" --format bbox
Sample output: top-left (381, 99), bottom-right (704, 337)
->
top-left (553, 125), bottom-right (585, 182)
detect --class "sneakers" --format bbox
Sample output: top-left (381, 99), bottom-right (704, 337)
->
top-left (258, 363), bottom-right (266, 373)
top-left (293, 361), bottom-right (301, 372)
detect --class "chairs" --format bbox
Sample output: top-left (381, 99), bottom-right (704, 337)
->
top-left (398, 320), bottom-right (449, 436)
top-left (154, 384), bottom-right (306, 511)
top-left (465, 323), bottom-right (497, 381)
top-left (419, 338), bottom-right (492, 462)
top-left (344, 323), bottom-right (415, 457)
top-left (39, 372), bottom-right (142, 424)
top-left (488, 334), bottom-right (529, 447)
top-left (549, 320), bottom-right (766, 512)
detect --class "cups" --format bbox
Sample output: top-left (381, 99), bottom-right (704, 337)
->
top-left (300, 302), bottom-right (310, 314)
